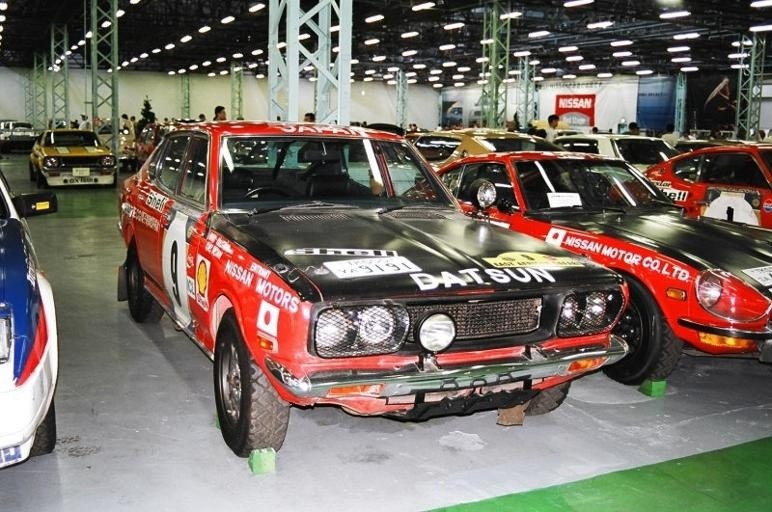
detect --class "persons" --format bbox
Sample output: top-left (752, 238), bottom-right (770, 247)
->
top-left (592, 122), bottom-right (765, 147)
top-left (213, 106), bottom-right (226, 121)
top-left (545, 115), bottom-right (559, 144)
top-left (304, 113), bottom-right (315, 122)
top-left (398, 120), bottom-right (480, 134)
top-left (70, 112), bottom-right (207, 160)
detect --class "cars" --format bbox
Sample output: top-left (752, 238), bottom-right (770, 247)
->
top-left (642, 144), bottom-right (772, 235)
top-left (31, 129), bottom-right (121, 191)
top-left (397, 128), bottom-right (568, 181)
top-left (552, 133), bottom-right (683, 183)
top-left (2, 121), bottom-right (36, 152)
top-left (1, 168), bottom-right (62, 466)
top-left (94, 117), bottom-right (179, 170)
top-left (112, 118), bottom-right (628, 463)
top-left (398, 151), bottom-right (772, 386)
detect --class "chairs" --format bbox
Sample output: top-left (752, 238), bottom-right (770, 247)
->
top-left (221, 166), bottom-right (255, 203)
top-left (300, 149), bottom-right (352, 196)
